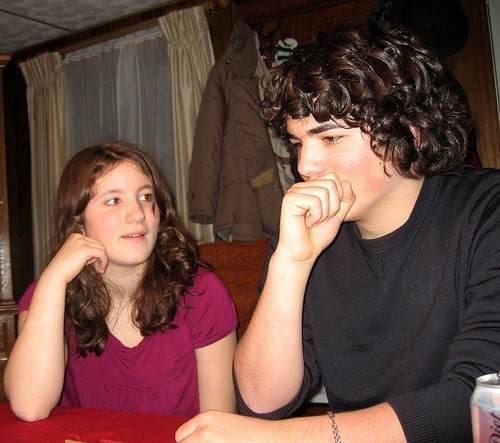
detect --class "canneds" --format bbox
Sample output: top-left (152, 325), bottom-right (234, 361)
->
top-left (470, 372), bottom-right (500, 443)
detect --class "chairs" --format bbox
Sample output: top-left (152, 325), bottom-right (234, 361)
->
top-left (197, 235), bottom-right (268, 345)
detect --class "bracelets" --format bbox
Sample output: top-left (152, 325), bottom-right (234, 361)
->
top-left (326, 410), bottom-right (341, 443)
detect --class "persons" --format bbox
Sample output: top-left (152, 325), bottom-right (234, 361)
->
top-left (175, 19), bottom-right (500, 443)
top-left (3, 139), bottom-right (239, 422)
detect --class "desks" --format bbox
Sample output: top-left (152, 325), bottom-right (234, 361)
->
top-left (0, 402), bottom-right (192, 443)
